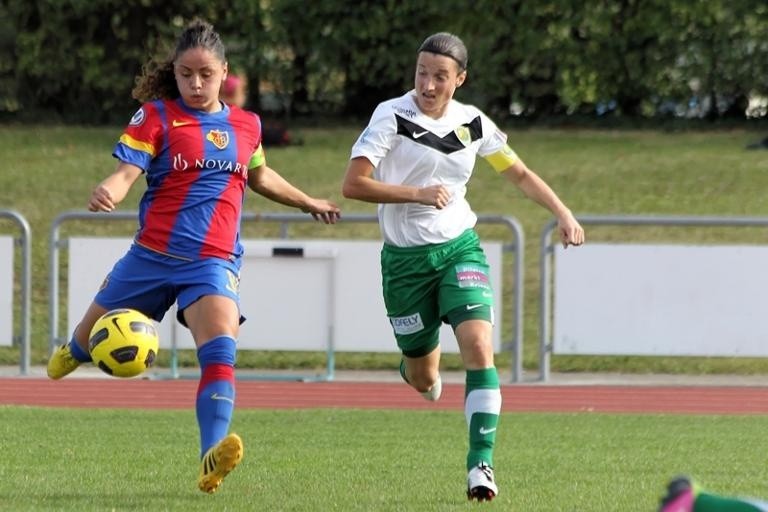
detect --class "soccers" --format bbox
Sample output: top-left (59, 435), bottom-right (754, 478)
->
top-left (89, 308), bottom-right (160, 377)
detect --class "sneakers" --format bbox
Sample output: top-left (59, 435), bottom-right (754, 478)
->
top-left (198, 433), bottom-right (243, 494)
top-left (466, 460), bottom-right (498, 503)
top-left (46, 341), bottom-right (81, 380)
top-left (656, 479), bottom-right (702, 512)
top-left (421, 375), bottom-right (442, 402)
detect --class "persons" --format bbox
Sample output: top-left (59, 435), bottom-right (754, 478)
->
top-left (343, 31), bottom-right (586, 501)
top-left (45, 13), bottom-right (342, 494)
top-left (217, 74), bottom-right (248, 109)
